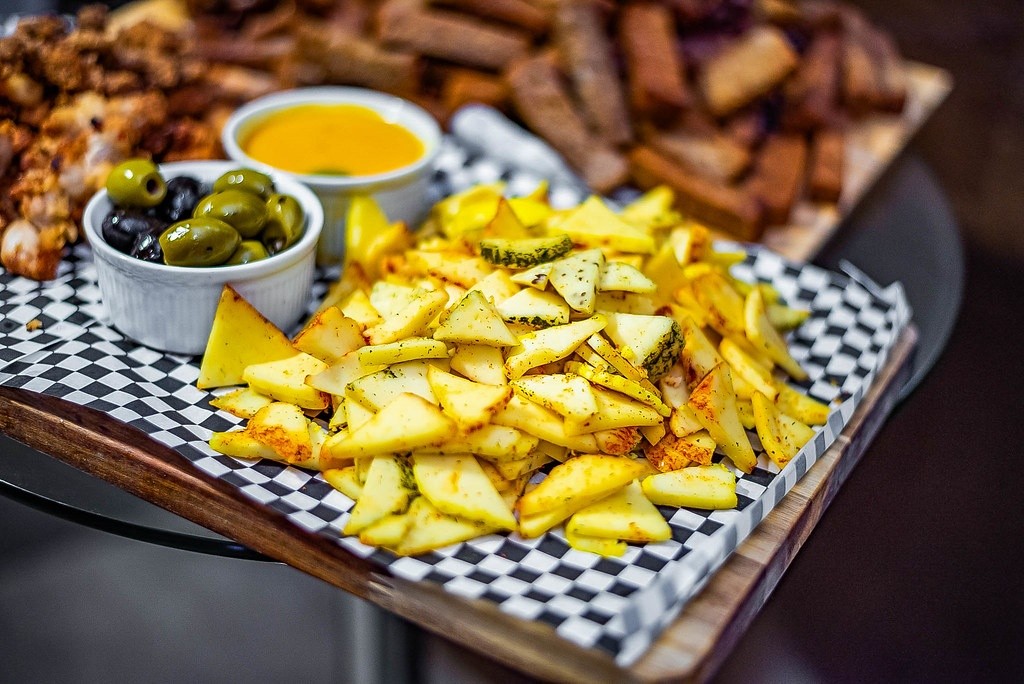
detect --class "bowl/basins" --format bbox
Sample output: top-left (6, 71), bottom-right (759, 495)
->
top-left (84, 157), bottom-right (326, 356)
top-left (219, 88), bottom-right (447, 265)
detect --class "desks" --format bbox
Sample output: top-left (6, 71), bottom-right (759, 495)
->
top-left (0, 0), bottom-right (971, 684)
